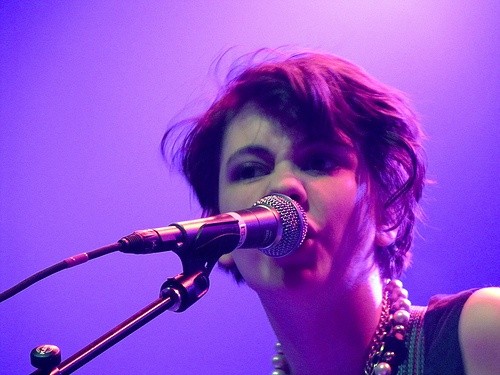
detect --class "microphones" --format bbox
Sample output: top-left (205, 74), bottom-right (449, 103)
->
top-left (120, 193), bottom-right (307, 258)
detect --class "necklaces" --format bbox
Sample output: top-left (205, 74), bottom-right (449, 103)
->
top-left (270, 276), bottom-right (427, 375)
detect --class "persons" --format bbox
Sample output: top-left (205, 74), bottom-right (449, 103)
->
top-left (158, 40), bottom-right (498, 374)
